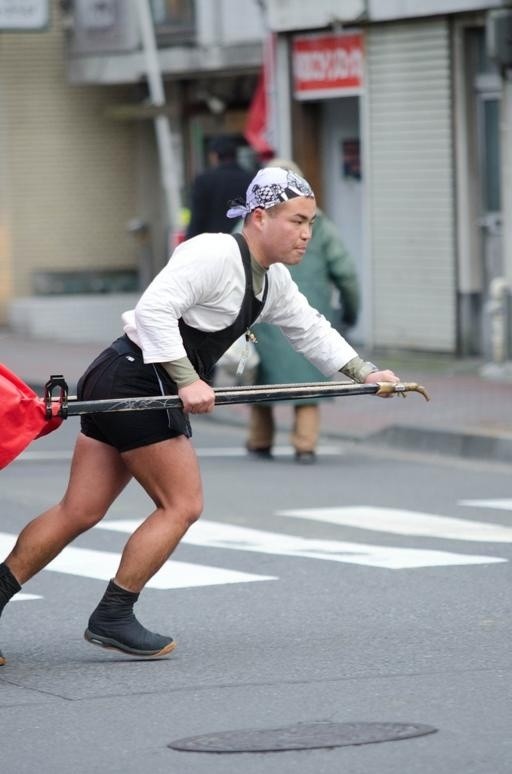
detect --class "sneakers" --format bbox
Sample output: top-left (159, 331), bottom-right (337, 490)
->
top-left (244, 440), bottom-right (271, 459)
top-left (294, 451), bottom-right (317, 464)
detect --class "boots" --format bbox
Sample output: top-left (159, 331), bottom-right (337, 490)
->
top-left (1, 562), bottom-right (23, 666)
top-left (83, 578), bottom-right (177, 658)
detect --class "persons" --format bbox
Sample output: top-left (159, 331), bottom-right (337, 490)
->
top-left (1, 168), bottom-right (400, 669)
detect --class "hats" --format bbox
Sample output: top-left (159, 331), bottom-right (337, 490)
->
top-left (225, 166), bottom-right (315, 219)
